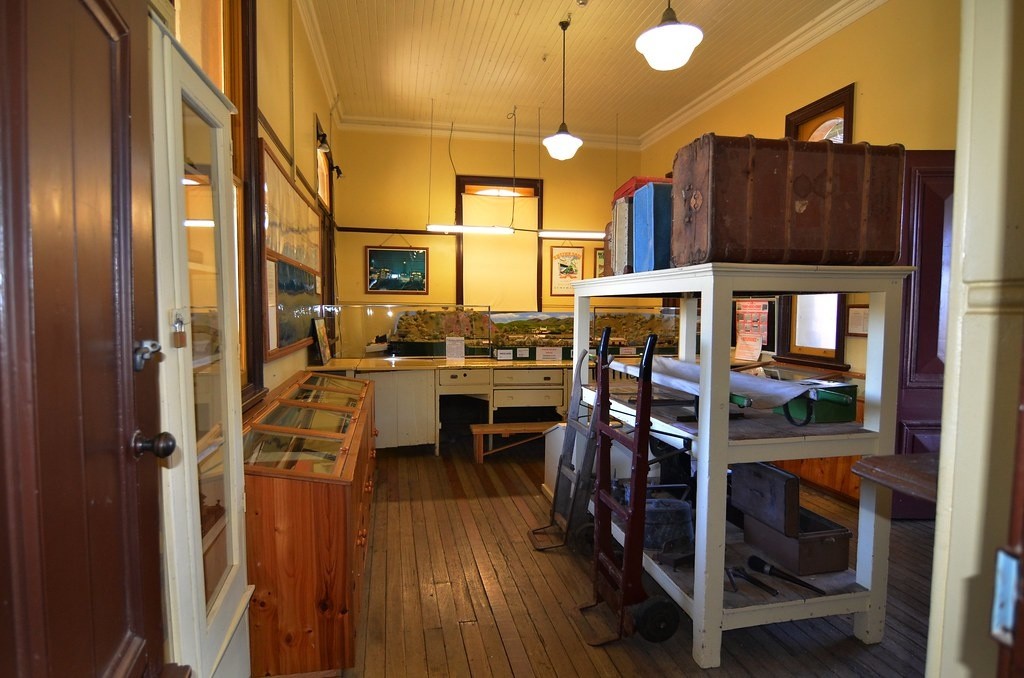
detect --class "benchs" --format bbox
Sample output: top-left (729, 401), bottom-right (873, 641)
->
top-left (470, 422), bottom-right (561, 464)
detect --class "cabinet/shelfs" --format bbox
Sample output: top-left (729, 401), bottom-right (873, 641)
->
top-left (306, 357), bottom-right (643, 457)
top-left (541, 264), bottom-right (918, 669)
top-left (0, 0), bottom-right (257, 678)
top-left (242, 371), bottom-right (378, 678)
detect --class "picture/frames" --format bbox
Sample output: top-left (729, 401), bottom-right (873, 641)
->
top-left (311, 317), bottom-right (331, 367)
top-left (550, 246), bottom-right (585, 296)
top-left (364, 245), bottom-right (429, 296)
top-left (594, 248), bottom-right (605, 278)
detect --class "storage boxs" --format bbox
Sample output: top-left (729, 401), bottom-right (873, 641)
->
top-left (604, 131), bottom-right (907, 269)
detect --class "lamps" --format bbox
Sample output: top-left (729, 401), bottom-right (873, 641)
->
top-left (538, 109), bottom-right (620, 240)
top-left (542, 22), bottom-right (584, 160)
top-left (635, 0), bottom-right (703, 72)
top-left (426, 98), bottom-right (517, 235)
